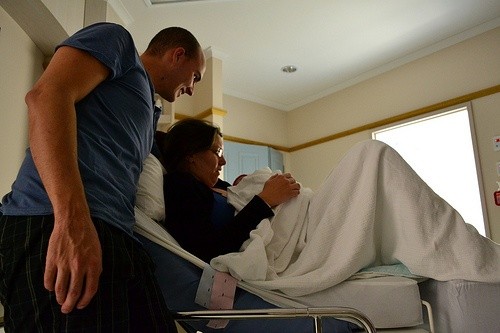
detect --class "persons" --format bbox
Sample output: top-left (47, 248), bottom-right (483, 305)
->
top-left (150, 118), bottom-right (500, 285)
top-left (1, 21), bottom-right (208, 332)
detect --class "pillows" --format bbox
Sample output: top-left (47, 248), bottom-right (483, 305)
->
top-left (133, 153), bottom-right (168, 222)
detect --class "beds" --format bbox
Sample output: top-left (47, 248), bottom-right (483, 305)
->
top-left (131, 206), bottom-right (499, 332)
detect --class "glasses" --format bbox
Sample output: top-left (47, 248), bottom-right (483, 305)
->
top-left (201, 145), bottom-right (224, 157)
